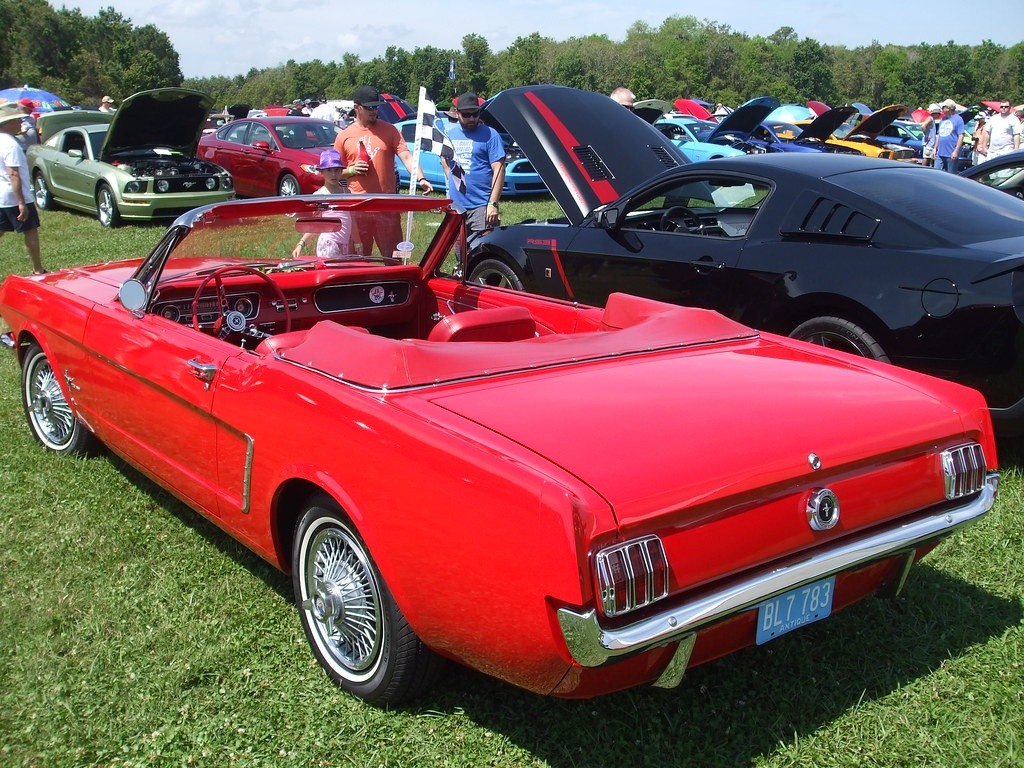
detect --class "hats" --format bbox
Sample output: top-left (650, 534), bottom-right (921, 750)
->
top-left (457, 92), bottom-right (480, 110)
top-left (940, 99), bottom-right (956, 110)
top-left (17, 98), bottom-right (35, 109)
top-left (293, 99), bottom-right (304, 106)
top-left (354, 87), bottom-right (386, 107)
top-left (102, 96), bottom-right (114, 103)
top-left (1014, 104), bottom-right (1024, 110)
top-left (305, 99), bottom-right (311, 104)
top-left (315, 149), bottom-right (347, 171)
top-left (929, 103), bottom-right (941, 114)
top-left (974, 112), bottom-right (985, 119)
top-left (0, 102), bottom-right (30, 124)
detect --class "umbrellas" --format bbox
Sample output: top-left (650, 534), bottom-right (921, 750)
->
top-left (0, 84), bottom-right (72, 111)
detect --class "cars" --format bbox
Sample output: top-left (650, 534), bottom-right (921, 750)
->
top-left (249, 107), bottom-right (318, 141)
top-left (629, 96), bottom-right (1023, 170)
top-left (1, 195), bottom-right (1000, 698)
top-left (195, 115), bottom-right (344, 198)
top-left (957, 150), bottom-right (1024, 199)
top-left (376, 94), bottom-right (551, 198)
top-left (25, 86), bottom-right (236, 228)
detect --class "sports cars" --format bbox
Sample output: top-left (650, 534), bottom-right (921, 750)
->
top-left (459, 84), bottom-right (1024, 422)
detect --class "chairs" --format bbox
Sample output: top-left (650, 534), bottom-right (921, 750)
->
top-left (255, 326), bottom-right (370, 357)
top-left (293, 128), bottom-right (312, 146)
top-left (427, 306), bottom-right (536, 342)
top-left (661, 129), bottom-right (672, 139)
top-left (256, 133), bottom-right (271, 143)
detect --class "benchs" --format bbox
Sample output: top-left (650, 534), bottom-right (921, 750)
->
top-left (230, 131), bottom-right (291, 145)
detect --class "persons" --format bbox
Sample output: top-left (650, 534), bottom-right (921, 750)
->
top-left (714, 103), bottom-right (724, 122)
top-left (0, 86), bottom-right (434, 348)
top-left (917, 99), bottom-right (1022, 183)
top-left (439, 92), bottom-right (506, 262)
top-left (610, 86), bottom-right (636, 113)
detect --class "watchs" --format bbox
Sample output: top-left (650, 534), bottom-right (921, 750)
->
top-left (488, 201), bottom-right (499, 207)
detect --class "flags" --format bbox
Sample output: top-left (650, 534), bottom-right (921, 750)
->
top-left (421, 92), bottom-right (467, 197)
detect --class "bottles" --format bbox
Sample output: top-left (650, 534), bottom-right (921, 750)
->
top-left (358, 140), bottom-right (371, 175)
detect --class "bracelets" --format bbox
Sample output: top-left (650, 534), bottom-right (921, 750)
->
top-left (933, 149), bottom-right (937, 151)
top-left (418, 178), bottom-right (429, 182)
top-left (297, 240), bottom-right (306, 248)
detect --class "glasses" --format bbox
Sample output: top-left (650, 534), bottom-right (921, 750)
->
top-left (623, 105), bottom-right (635, 112)
top-left (458, 111), bottom-right (479, 118)
top-left (1000, 106), bottom-right (1009, 108)
top-left (363, 105), bottom-right (380, 111)
